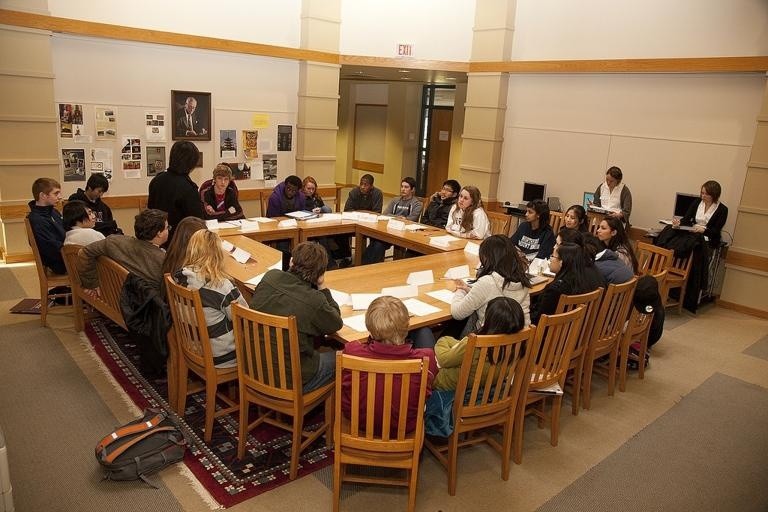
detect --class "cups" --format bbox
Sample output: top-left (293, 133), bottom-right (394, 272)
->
top-left (673, 216), bottom-right (680, 227)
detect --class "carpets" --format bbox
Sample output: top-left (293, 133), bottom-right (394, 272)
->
top-left (83, 313), bottom-right (334, 509)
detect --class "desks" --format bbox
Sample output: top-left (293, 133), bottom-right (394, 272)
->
top-left (272, 210), bottom-right (374, 268)
top-left (355, 217), bottom-right (484, 266)
top-left (216, 220), bottom-right (300, 253)
top-left (645, 220), bottom-right (728, 313)
top-left (507, 207), bottom-right (527, 230)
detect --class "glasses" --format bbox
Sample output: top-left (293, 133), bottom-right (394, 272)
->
top-left (550, 254), bottom-right (562, 261)
top-left (162, 225), bottom-right (172, 230)
top-left (442, 187), bottom-right (454, 192)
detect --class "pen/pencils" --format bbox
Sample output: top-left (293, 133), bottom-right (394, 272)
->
top-left (432, 230), bottom-right (440, 232)
top-left (416, 229), bottom-right (429, 232)
top-left (439, 278), bottom-right (454, 280)
top-left (587, 197), bottom-right (592, 204)
top-left (316, 210), bottom-right (319, 212)
top-left (409, 314), bottom-right (415, 318)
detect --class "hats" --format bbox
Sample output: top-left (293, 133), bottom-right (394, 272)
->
top-left (634, 274), bottom-right (660, 313)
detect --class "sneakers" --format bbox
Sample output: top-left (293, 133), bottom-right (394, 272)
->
top-left (328, 260), bottom-right (338, 269)
top-left (339, 257), bottom-right (352, 267)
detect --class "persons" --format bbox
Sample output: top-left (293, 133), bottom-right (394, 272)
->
top-left (361, 177), bottom-right (424, 264)
top-left (76, 210), bottom-right (173, 319)
top-left (524, 241), bottom-right (587, 364)
top-left (553, 232), bottom-right (606, 306)
top-left (172, 228), bottom-right (251, 369)
top-left (509, 201), bottom-right (555, 259)
top-left (63, 200), bottom-right (106, 245)
top-left (672, 181), bottom-right (729, 297)
top-left (418, 298), bottom-right (530, 406)
top-left (405, 180), bottom-right (462, 261)
top-left (62, 174), bottom-right (124, 237)
top-left (560, 205), bottom-right (595, 242)
top-left (174, 96), bottom-right (206, 136)
top-left (162, 217), bottom-right (210, 324)
top-left (340, 295), bottom-right (439, 441)
top-left (147, 141), bottom-right (203, 253)
top-left (596, 216), bottom-right (635, 280)
top-left (243, 243), bottom-right (344, 393)
top-left (450, 233), bottom-right (532, 341)
top-left (339, 174), bottom-right (383, 267)
top-left (300, 176), bottom-right (336, 266)
top-left (266, 176), bottom-right (308, 271)
top-left (445, 186), bottom-right (490, 237)
top-left (585, 167), bottom-right (632, 236)
top-left (584, 238), bottom-right (638, 338)
top-left (199, 165), bottom-right (243, 223)
top-left (28, 178), bottom-right (73, 278)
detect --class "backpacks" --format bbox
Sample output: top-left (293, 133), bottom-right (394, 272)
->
top-left (94, 409), bottom-right (189, 483)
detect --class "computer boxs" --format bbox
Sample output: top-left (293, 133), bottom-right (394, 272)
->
top-left (506, 204), bottom-right (528, 217)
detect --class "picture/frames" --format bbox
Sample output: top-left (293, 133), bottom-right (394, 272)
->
top-left (146, 146), bottom-right (166, 176)
top-left (583, 192), bottom-right (595, 212)
top-left (171, 89), bottom-right (211, 141)
top-left (197, 152), bottom-right (203, 168)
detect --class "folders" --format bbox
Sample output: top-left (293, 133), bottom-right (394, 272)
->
top-left (284, 210), bottom-right (320, 222)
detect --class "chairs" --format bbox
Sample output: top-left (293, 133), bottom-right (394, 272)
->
top-left (595, 271), bottom-right (668, 392)
top-left (333, 351), bottom-right (430, 512)
top-left (486, 211), bottom-right (512, 237)
top-left (541, 287), bottom-right (605, 429)
top-left (634, 240), bottom-right (674, 297)
top-left (419, 198), bottom-right (429, 224)
top-left (24, 212), bottom-right (102, 328)
top-left (648, 226), bottom-right (698, 315)
top-left (566, 276), bottom-right (640, 410)
top-left (424, 324), bottom-right (536, 496)
top-left (498, 304), bottom-right (587, 464)
top-left (230, 300), bottom-right (335, 480)
top-left (260, 192), bottom-right (293, 252)
top-left (586, 212), bottom-right (606, 237)
top-left (163, 272), bottom-right (263, 442)
top-left (547, 210), bottom-right (566, 237)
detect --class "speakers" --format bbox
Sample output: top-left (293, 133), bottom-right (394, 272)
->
top-left (548, 197), bottom-right (559, 211)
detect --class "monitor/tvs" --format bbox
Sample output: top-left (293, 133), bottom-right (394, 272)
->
top-left (583, 192), bottom-right (596, 212)
top-left (523, 182), bottom-right (547, 202)
top-left (672, 192), bottom-right (702, 217)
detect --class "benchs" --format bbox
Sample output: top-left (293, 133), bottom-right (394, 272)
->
top-left (59, 244), bottom-right (193, 413)
